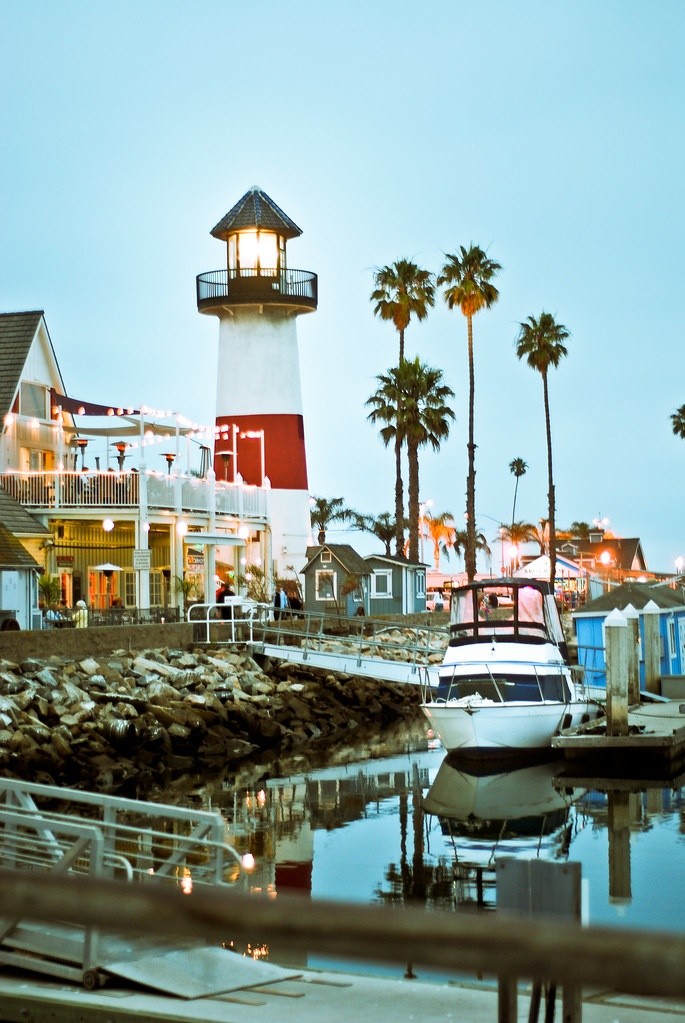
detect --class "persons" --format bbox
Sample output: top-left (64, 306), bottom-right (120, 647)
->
top-left (434, 588), bottom-right (443, 612)
top-left (46, 602), bottom-right (61, 620)
top-left (489, 593), bottom-right (498, 608)
top-left (39, 601), bottom-right (47, 617)
top-left (60, 600), bottom-right (69, 612)
top-left (110, 598), bottom-right (125, 614)
top-left (217, 582), bottom-right (235, 619)
top-left (275, 588), bottom-right (301, 620)
top-left (73, 601), bottom-right (88, 628)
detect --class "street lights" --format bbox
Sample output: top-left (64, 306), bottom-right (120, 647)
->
top-left (419, 499), bottom-right (434, 563)
top-left (600, 551), bottom-right (610, 594)
top-left (676, 557), bottom-right (684, 576)
top-left (499, 525), bottom-right (507, 577)
top-left (509, 546), bottom-right (517, 579)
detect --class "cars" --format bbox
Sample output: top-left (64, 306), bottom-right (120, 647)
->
top-left (426, 592), bottom-right (451, 612)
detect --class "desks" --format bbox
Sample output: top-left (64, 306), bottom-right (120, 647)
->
top-left (44, 620), bottom-right (77, 628)
top-left (44, 485), bottom-right (55, 507)
top-left (84, 483), bottom-right (126, 504)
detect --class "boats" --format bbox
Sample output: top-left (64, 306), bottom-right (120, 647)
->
top-left (421, 754), bottom-right (589, 870)
top-left (417, 577), bottom-right (605, 760)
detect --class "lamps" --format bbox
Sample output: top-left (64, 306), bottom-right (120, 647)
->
top-left (95, 562), bottom-right (123, 577)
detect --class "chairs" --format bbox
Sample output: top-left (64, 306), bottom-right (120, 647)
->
top-left (1, 473), bottom-right (265, 519)
